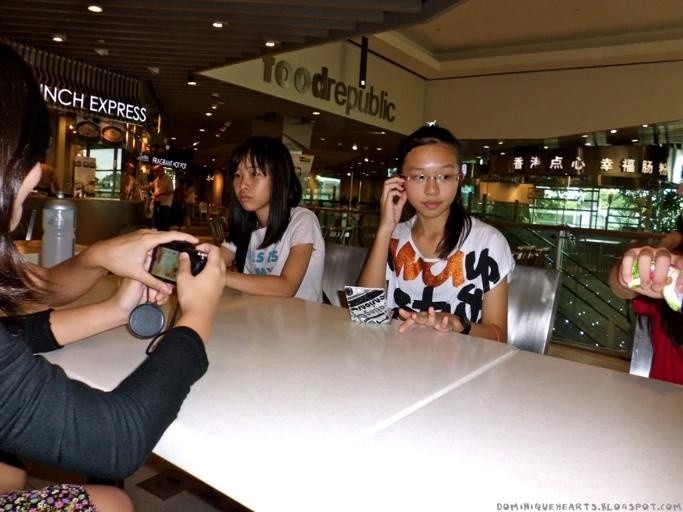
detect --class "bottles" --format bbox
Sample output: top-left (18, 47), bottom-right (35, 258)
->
top-left (40, 190), bottom-right (77, 269)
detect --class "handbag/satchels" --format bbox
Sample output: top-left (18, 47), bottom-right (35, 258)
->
top-left (128, 183), bottom-right (154, 219)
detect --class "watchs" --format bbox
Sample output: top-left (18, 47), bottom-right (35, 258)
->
top-left (455, 313), bottom-right (471, 335)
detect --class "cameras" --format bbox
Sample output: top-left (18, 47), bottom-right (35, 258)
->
top-left (147, 242), bottom-right (206, 288)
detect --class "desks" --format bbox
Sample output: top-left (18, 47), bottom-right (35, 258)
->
top-left (19, 237), bottom-right (683, 512)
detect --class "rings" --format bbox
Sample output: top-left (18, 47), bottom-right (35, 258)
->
top-left (152, 228), bottom-right (157, 232)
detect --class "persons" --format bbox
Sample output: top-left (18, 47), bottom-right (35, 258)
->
top-left (608, 210), bottom-right (683, 385)
top-left (142, 164), bottom-right (175, 231)
top-left (17, 228), bottom-right (200, 307)
top-left (351, 196), bottom-right (358, 209)
top-left (0, 43), bottom-right (226, 512)
top-left (217, 135), bottom-right (325, 304)
top-left (355, 125), bottom-right (516, 343)
top-left (340, 196), bottom-right (349, 210)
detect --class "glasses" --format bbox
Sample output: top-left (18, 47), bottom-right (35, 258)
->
top-left (398, 173), bottom-right (461, 184)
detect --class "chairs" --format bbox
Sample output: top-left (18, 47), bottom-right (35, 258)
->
top-left (323, 240), bottom-right (371, 309)
top-left (505, 261), bottom-right (561, 356)
